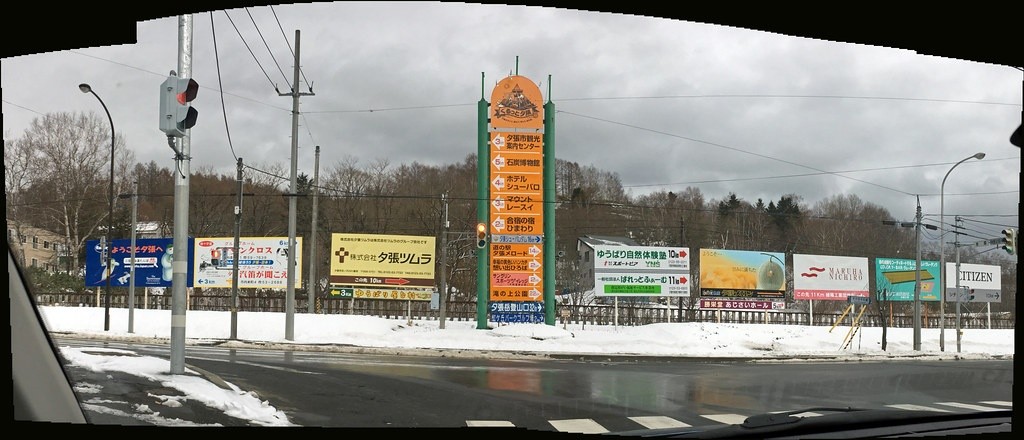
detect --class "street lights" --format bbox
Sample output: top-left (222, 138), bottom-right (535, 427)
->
top-left (940, 153), bottom-right (985, 351)
top-left (78, 84), bottom-right (115, 329)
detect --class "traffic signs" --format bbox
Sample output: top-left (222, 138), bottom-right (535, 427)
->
top-left (194, 236), bottom-right (303, 289)
top-left (488, 73), bottom-right (543, 323)
top-left (946, 262), bottom-right (1002, 303)
top-left (327, 275), bottom-right (437, 302)
top-left (590, 245), bottom-right (690, 298)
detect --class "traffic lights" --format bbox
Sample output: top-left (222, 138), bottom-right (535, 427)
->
top-left (1002, 229), bottom-right (1015, 255)
top-left (159, 74), bottom-right (199, 136)
top-left (475, 224), bottom-right (487, 248)
top-left (964, 288), bottom-right (975, 302)
